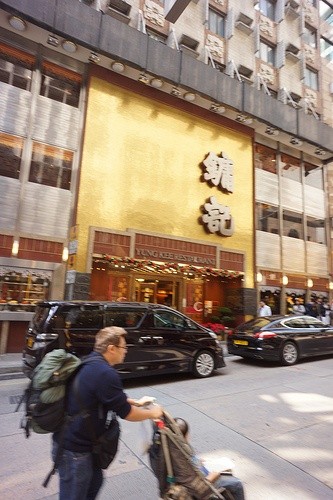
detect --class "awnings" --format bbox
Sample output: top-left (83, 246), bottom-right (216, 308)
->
top-left (260, 285), bottom-right (328, 299)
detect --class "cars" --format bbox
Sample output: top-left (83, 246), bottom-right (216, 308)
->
top-left (227, 314), bottom-right (333, 365)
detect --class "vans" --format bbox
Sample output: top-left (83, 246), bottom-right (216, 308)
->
top-left (22, 299), bottom-right (227, 382)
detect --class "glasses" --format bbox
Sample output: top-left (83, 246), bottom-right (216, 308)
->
top-left (115, 345), bottom-right (128, 351)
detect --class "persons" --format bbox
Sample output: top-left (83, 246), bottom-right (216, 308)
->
top-left (172, 417), bottom-right (245, 500)
top-left (50, 325), bottom-right (164, 500)
top-left (293, 295), bottom-right (333, 325)
top-left (258, 295), bottom-right (272, 317)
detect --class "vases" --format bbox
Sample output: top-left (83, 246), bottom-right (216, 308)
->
top-left (216, 335), bottom-right (222, 341)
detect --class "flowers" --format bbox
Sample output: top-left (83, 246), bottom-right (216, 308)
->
top-left (201, 322), bottom-right (225, 335)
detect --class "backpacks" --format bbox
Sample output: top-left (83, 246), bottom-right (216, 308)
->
top-left (15, 349), bottom-right (106, 438)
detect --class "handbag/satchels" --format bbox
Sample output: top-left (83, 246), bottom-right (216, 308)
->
top-left (91, 419), bottom-right (120, 469)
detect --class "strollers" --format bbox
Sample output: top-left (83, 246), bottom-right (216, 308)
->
top-left (144, 396), bottom-right (234, 500)
